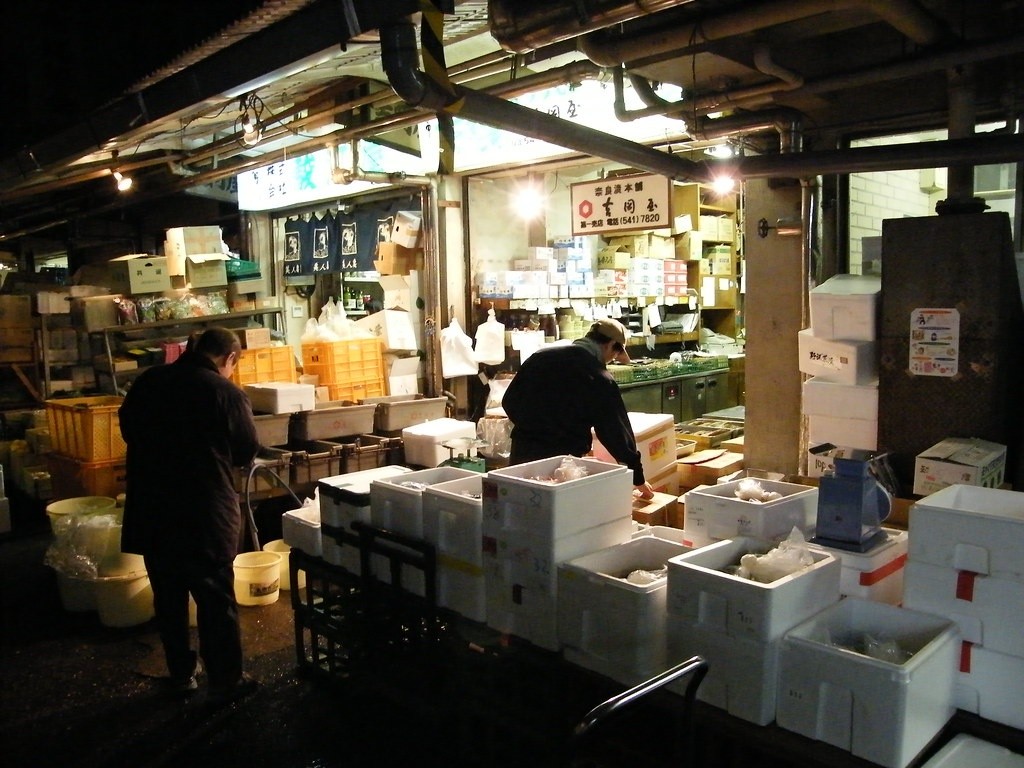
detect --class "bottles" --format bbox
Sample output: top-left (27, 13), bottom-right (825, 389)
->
top-left (344, 286), bottom-right (370, 311)
top-left (497, 314), bottom-right (548, 338)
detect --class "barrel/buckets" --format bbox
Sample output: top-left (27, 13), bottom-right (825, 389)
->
top-left (264, 539), bottom-right (306, 591)
top-left (45, 497), bottom-right (152, 628)
top-left (232, 552), bottom-right (281, 605)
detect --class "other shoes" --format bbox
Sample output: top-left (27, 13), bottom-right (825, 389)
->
top-left (173, 659), bottom-right (202, 691)
top-left (206, 670), bottom-right (252, 702)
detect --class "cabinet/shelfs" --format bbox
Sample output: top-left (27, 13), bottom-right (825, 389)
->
top-left (672, 183), bottom-right (739, 349)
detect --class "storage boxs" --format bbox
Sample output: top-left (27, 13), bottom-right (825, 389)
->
top-left (1, 210), bottom-right (1024, 768)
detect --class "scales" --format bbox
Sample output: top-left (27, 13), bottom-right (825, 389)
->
top-left (437, 437), bottom-right (489, 473)
top-left (808, 444), bottom-right (892, 553)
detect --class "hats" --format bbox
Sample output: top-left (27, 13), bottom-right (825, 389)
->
top-left (592, 319), bottom-right (631, 364)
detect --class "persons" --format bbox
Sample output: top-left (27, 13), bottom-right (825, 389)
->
top-left (118, 325), bottom-right (263, 686)
top-left (501, 317), bottom-right (654, 499)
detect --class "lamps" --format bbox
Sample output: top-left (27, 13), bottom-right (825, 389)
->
top-left (111, 168), bottom-right (131, 192)
top-left (241, 116), bottom-right (262, 147)
